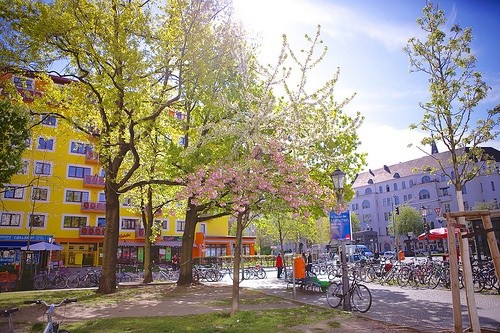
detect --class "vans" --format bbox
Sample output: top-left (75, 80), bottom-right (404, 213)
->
top-left (338, 244), bottom-right (373, 262)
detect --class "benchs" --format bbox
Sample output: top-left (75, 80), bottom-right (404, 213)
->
top-left (286, 277), bottom-right (330, 293)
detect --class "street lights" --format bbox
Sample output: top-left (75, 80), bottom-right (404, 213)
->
top-left (329, 168), bottom-right (352, 313)
top-left (407, 231), bottom-right (414, 257)
top-left (419, 205), bottom-right (433, 272)
top-left (48, 235), bottom-right (54, 274)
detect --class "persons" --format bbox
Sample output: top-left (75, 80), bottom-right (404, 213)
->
top-left (302, 251), bottom-right (312, 272)
top-left (326, 250), bottom-right (332, 258)
top-left (172, 254), bottom-right (177, 271)
top-left (276, 253), bottom-right (283, 278)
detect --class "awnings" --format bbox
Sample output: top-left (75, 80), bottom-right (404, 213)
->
top-left (117, 240), bottom-right (197, 248)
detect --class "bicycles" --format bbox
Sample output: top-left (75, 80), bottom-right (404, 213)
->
top-left (116, 264), bottom-right (145, 283)
top-left (239, 261), bottom-right (266, 283)
top-left (325, 270), bottom-right (373, 313)
top-left (306, 250), bottom-right (500, 298)
top-left (23, 297), bottom-right (78, 333)
top-left (0, 307), bottom-right (21, 333)
top-left (192, 262), bottom-right (233, 283)
top-left (151, 264), bottom-right (179, 282)
top-left (33, 268), bottom-right (102, 290)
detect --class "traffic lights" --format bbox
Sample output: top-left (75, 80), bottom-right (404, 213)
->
top-left (395, 206), bottom-right (400, 215)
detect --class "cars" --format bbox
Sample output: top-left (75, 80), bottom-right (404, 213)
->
top-left (384, 251), bottom-right (396, 260)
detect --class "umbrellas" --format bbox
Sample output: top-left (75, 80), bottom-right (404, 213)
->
top-left (417, 226), bottom-right (458, 253)
top-left (21, 241), bottom-right (64, 270)
